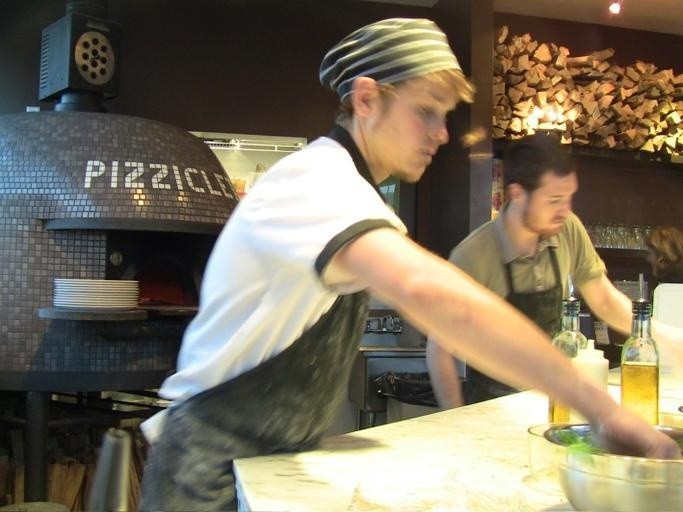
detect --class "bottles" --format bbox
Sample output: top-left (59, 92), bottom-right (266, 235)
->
top-left (570, 338), bottom-right (610, 425)
top-left (621, 296), bottom-right (660, 423)
top-left (548, 296), bottom-right (588, 423)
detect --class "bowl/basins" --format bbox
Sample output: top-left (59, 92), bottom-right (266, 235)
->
top-left (544, 423), bottom-right (683, 512)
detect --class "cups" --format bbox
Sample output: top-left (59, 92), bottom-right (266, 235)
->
top-left (528, 424), bottom-right (555, 481)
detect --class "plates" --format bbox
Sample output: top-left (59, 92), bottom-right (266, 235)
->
top-left (52, 277), bottom-right (140, 312)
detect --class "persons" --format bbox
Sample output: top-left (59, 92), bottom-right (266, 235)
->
top-left (135, 17), bottom-right (683, 511)
top-left (423, 131), bottom-right (632, 409)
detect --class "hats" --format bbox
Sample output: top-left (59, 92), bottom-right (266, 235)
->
top-left (319, 18), bottom-right (462, 100)
